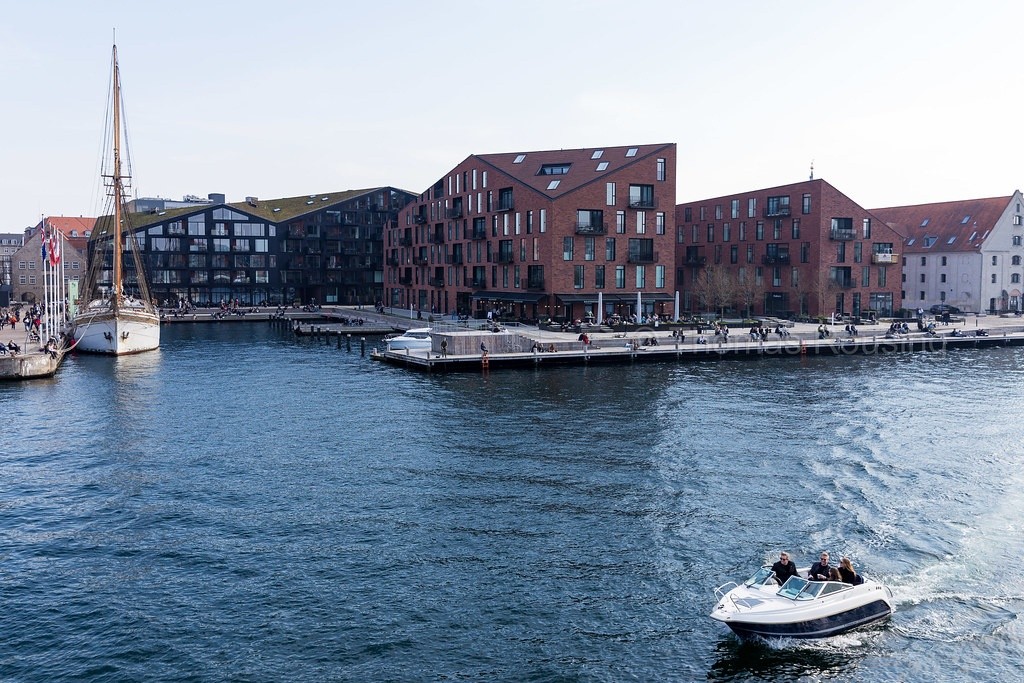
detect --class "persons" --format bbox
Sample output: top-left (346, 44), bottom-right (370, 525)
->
top-left (0, 294), bottom-right (997, 358)
top-left (808, 552), bottom-right (833, 583)
top-left (769, 552), bottom-right (801, 585)
top-left (836, 557), bottom-right (857, 586)
top-left (826, 567), bottom-right (842, 584)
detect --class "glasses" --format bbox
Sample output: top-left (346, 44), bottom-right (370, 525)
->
top-left (820, 557), bottom-right (828, 561)
top-left (840, 561), bottom-right (843, 563)
top-left (780, 557), bottom-right (788, 561)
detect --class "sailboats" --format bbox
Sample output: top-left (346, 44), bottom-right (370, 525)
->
top-left (72, 28), bottom-right (160, 356)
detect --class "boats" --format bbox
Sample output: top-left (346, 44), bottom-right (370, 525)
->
top-left (383, 328), bottom-right (432, 349)
top-left (709, 562), bottom-right (895, 643)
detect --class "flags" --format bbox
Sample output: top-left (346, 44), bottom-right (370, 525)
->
top-left (40, 220), bottom-right (47, 261)
top-left (55, 233), bottom-right (60, 265)
top-left (48, 228), bottom-right (56, 266)
top-left (53, 232), bottom-right (56, 254)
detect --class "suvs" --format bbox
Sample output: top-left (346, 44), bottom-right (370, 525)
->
top-left (930, 305), bottom-right (960, 314)
